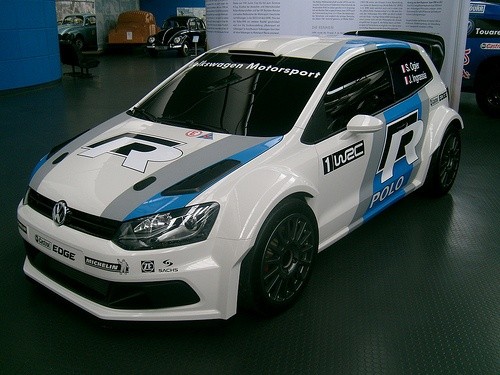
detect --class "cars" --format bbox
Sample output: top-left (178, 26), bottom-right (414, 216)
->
top-left (145, 15), bottom-right (206, 58)
top-left (16, 31), bottom-right (466, 325)
top-left (58, 12), bottom-right (97, 51)
top-left (455, 0), bottom-right (499, 112)
